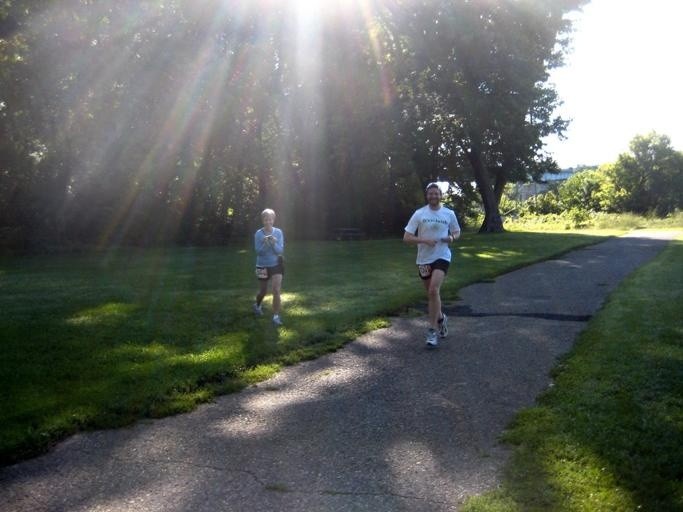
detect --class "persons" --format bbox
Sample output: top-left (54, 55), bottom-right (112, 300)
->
top-left (402, 183), bottom-right (460, 347)
top-left (253, 207), bottom-right (285, 327)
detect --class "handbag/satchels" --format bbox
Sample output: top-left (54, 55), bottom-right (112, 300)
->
top-left (278, 255), bottom-right (284, 265)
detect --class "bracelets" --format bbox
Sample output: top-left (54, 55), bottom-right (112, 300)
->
top-left (450, 234), bottom-right (457, 243)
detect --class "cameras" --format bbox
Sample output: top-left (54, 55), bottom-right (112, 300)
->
top-left (268, 235), bottom-right (273, 241)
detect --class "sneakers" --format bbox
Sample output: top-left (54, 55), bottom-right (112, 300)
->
top-left (425, 331), bottom-right (439, 346)
top-left (272, 314), bottom-right (283, 325)
top-left (438, 313), bottom-right (449, 338)
top-left (253, 303), bottom-right (264, 316)
